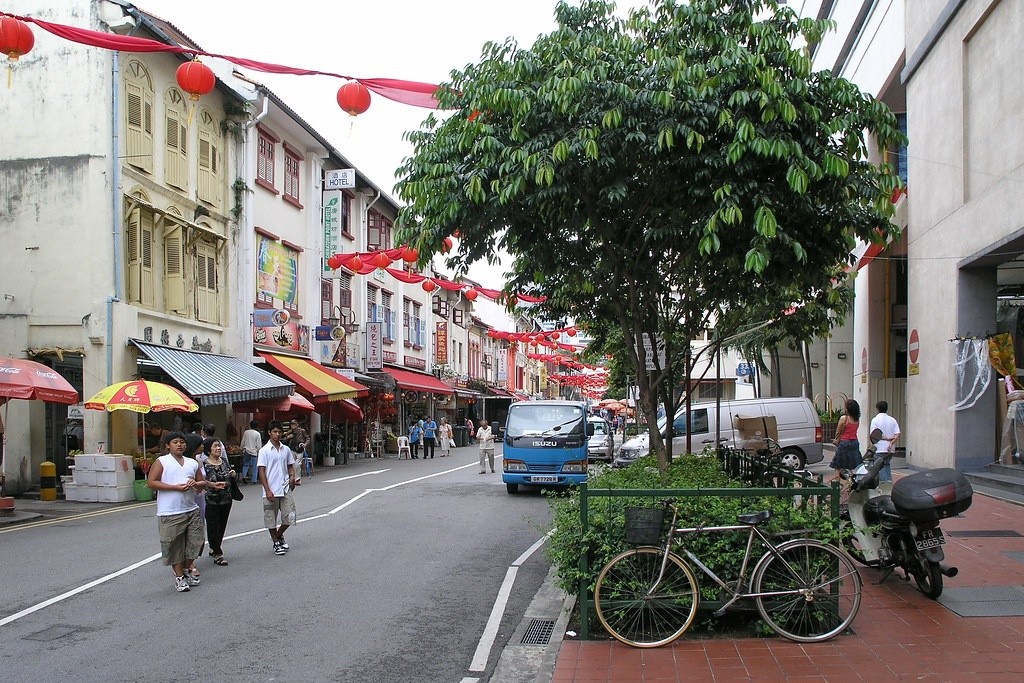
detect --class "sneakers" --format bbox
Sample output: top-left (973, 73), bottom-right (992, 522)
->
top-left (278, 535), bottom-right (289, 551)
top-left (273, 541), bottom-right (285, 554)
top-left (183, 569), bottom-right (200, 585)
top-left (175, 575), bottom-right (190, 592)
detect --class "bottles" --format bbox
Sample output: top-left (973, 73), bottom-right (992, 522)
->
top-left (1005, 373), bottom-right (1015, 392)
top-left (998, 299), bottom-right (1020, 322)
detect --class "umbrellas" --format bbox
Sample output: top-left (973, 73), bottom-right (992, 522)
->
top-left (232, 392), bottom-right (314, 420)
top-left (313, 397), bottom-right (364, 458)
top-left (599, 399), bottom-right (625, 415)
top-left (0, 354), bottom-right (77, 494)
top-left (84, 377), bottom-right (199, 481)
top-left (299, 443), bottom-right (312, 480)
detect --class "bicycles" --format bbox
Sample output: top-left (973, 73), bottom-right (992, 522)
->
top-left (592, 495), bottom-right (866, 649)
top-left (757, 438), bottom-right (783, 467)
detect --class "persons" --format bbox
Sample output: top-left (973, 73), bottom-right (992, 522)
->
top-left (257, 420), bottom-right (296, 556)
top-left (145, 422), bottom-right (235, 592)
top-left (283, 419), bottom-right (311, 486)
top-left (657, 404), bottom-right (664, 419)
top-left (829, 399), bottom-right (862, 475)
top-left (476, 419), bottom-right (495, 474)
top-left (408, 414), bottom-right (452, 459)
top-left (613, 414), bottom-right (623, 435)
top-left (870, 401), bottom-right (900, 481)
top-left (464, 417), bottom-right (474, 444)
top-left (240, 419), bottom-right (262, 484)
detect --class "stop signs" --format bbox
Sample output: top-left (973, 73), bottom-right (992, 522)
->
top-left (862, 347), bottom-right (867, 373)
top-left (909, 328), bottom-right (918, 363)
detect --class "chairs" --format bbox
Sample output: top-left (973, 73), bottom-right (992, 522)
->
top-left (397, 436), bottom-right (411, 460)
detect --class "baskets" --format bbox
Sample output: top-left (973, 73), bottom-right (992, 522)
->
top-left (624, 507), bottom-right (664, 545)
top-left (824, 476), bottom-right (856, 519)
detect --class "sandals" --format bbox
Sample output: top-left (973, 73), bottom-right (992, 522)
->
top-left (209, 551), bottom-right (213, 556)
top-left (214, 557), bottom-right (227, 565)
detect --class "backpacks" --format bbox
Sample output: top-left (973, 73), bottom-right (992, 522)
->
top-left (60, 426), bottom-right (78, 447)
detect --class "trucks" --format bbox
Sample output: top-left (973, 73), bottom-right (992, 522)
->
top-left (491, 398), bottom-right (595, 494)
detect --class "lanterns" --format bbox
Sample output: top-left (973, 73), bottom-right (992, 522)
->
top-left (380, 393), bottom-right (395, 418)
top-left (348, 229), bottom-right (610, 396)
top-left (337, 78), bottom-right (372, 133)
top-left (176, 54), bottom-right (215, 126)
top-left (0, 16), bottom-right (34, 89)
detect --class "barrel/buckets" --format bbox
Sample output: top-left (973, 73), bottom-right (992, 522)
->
top-left (134, 480), bottom-right (153, 501)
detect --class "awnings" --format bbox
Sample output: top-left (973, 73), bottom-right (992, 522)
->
top-left (129, 339), bottom-right (296, 405)
top-left (255, 344), bottom-right (369, 403)
top-left (453, 386), bottom-right (482, 398)
top-left (507, 391), bottom-right (529, 401)
top-left (382, 367), bottom-right (453, 394)
top-left (489, 387), bottom-right (509, 395)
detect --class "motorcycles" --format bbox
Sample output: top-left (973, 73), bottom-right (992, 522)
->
top-left (824, 427), bottom-right (973, 600)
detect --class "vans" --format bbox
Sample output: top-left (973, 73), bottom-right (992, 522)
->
top-left (585, 416), bottom-right (615, 464)
top-left (615, 396), bottom-right (823, 471)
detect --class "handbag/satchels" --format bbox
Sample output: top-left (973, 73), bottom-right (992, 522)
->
top-left (447, 424), bottom-right (454, 438)
top-left (221, 456), bottom-right (243, 501)
top-left (833, 415), bottom-right (847, 446)
top-left (449, 438), bottom-right (456, 447)
top-left (470, 430), bottom-right (474, 436)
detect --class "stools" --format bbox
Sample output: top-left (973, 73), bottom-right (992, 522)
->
top-left (302, 458), bottom-right (315, 476)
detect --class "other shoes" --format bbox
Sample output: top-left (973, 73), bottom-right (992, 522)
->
top-left (189, 564), bottom-right (200, 577)
top-left (242, 476), bottom-right (248, 484)
top-left (492, 470), bottom-right (495, 473)
top-left (410, 453), bottom-right (449, 459)
top-left (479, 471), bottom-right (485, 474)
top-left (251, 482), bottom-right (258, 485)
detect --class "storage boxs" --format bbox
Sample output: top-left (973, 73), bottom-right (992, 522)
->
top-left (66, 454), bottom-right (136, 503)
top-left (733, 414), bottom-right (778, 454)
